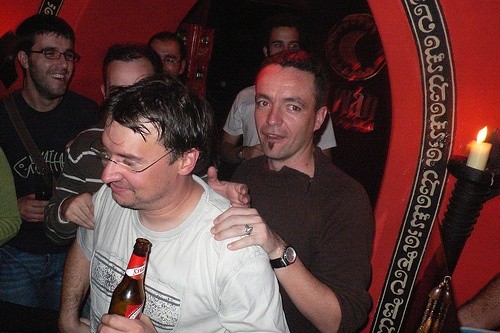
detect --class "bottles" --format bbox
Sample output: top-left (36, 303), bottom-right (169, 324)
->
top-left (108, 238), bottom-right (152, 319)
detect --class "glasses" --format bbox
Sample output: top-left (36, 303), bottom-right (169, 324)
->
top-left (31, 48), bottom-right (81, 61)
top-left (89, 136), bottom-right (174, 174)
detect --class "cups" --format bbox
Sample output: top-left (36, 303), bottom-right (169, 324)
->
top-left (32, 171), bottom-right (53, 202)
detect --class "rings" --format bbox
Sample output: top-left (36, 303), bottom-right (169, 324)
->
top-left (244, 223), bottom-right (253, 234)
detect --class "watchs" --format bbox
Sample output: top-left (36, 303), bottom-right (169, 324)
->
top-left (270, 243), bottom-right (297, 268)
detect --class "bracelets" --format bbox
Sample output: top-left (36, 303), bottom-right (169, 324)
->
top-left (239, 149), bottom-right (244, 159)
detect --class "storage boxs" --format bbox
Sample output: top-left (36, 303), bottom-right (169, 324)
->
top-left (175, 23), bottom-right (215, 96)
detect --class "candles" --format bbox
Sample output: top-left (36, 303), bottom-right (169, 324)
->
top-left (468, 142), bottom-right (492, 170)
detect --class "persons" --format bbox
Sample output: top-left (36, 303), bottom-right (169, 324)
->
top-left (221, 16), bottom-right (337, 160)
top-left (0, 15), bottom-right (100, 333)
top-left (210, 49), bottom-right (375, 333)
top-left (60, 73), bottom-right (291, 333)
top-left (42, 42), bottom-right (251, 319)
top-left (0, 147), bottom-right (22, 247)
top-left (149, 32), bottom-right (187, 78)
